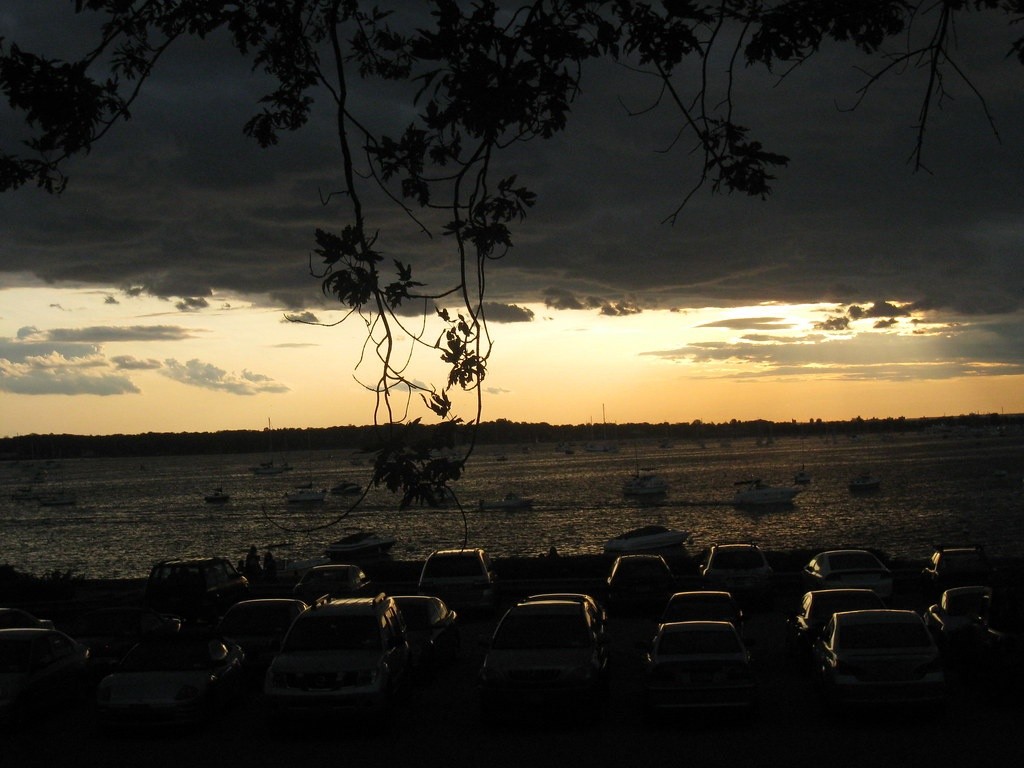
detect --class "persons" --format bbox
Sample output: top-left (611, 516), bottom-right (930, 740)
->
top-left (236, 544), bottom-right (278, 578)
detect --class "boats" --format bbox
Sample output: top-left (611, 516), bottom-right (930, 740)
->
top-left (324, 529), bottom-right (396, 564)
top-left (603, 525), bottom-right (688, 553)
top-left (14, 442), bottom-right (894, 513)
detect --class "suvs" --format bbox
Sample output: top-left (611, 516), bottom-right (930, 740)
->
top-left (264, 591), bottom-right (411, 730)
top-left (698, 543), bottom-right (783, 600)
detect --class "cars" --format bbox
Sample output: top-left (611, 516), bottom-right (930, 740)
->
top-left (391, 596), bottom-right (459, 677)
top-left (785, 588), bottom-right (887, 676)
top-left (643, 589), bottom-right (755, 724)
top-left (606, 555), bottom-right (682, 615)
top-left (293, 564), bottom-right (385, 602)
top-left (801, 548), bottom-right (905, 603)
top-left (416, 548), bottom-right (507, 621)
top-left (147, 558), bottom-right (251, 623)
top-left (811, 609), bottom-right (948, 718)
top-left (208, 597), bottom-right (307, 699)
top-left (0, 605), bottom-right (215, 727)
top-left (922, 543), bottom-right (1005, 655)
top-left (477, 593), bottom-right (611, 725)
